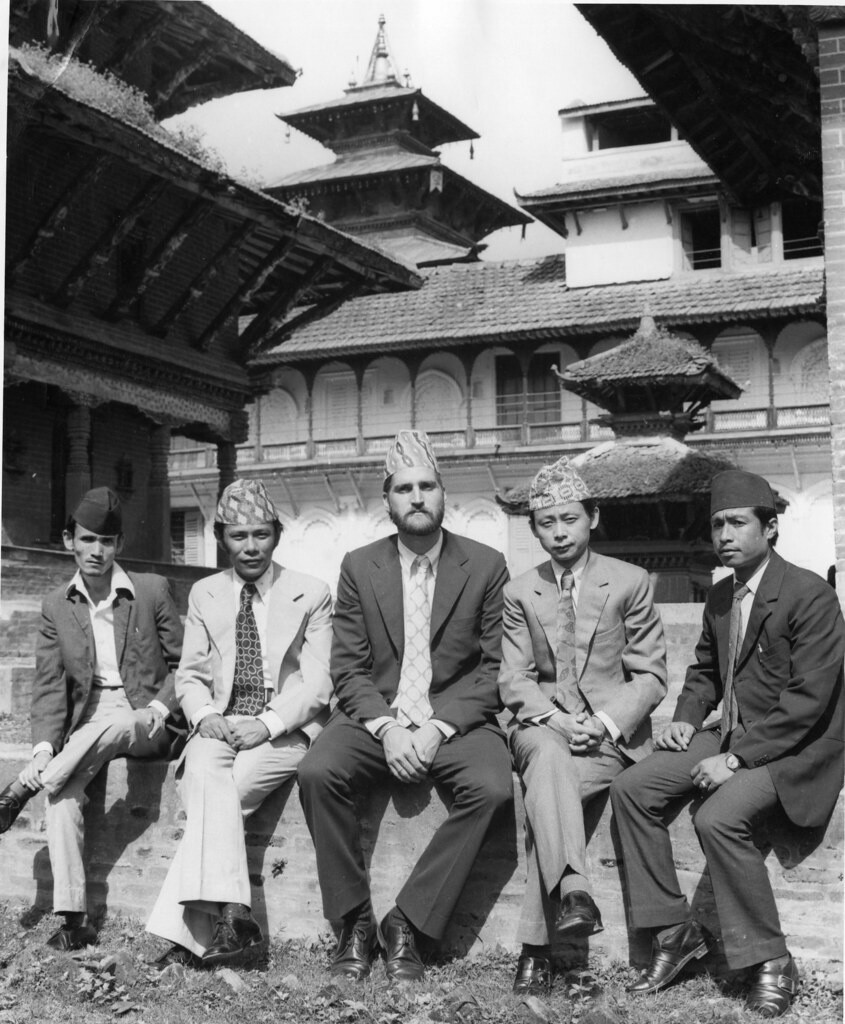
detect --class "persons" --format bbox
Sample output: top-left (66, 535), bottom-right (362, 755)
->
top-left (496, 455), bottom-right (667, 1001)
top-left (296, 430), bottom-right (511, 982)
top-left (144, 478), bottom-right (336, 968)
top-left (0, 487), bottom-right (193, 952)
top-left (611, 469), bottom-right (845, 1017)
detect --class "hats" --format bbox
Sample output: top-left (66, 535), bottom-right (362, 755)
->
top-left (710, 470), bottom-right (777, 516)
top-left (383, 429), bottom-right (441, 481)
top-left (529, 456), bottom-right (591, 511)
top-left (214, 479), bottom-right (279, 524)
top-left (71, 486), bottom-right (122, 535)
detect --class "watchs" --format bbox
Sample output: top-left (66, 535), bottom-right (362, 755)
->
top-left (726, 751), bottom-right (743, 772)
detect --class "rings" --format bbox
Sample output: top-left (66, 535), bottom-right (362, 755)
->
top-left (701, 780), bottom-right (708, 787)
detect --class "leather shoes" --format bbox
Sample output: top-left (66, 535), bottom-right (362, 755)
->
top-left (202, 913), bottom-right (264, 961)
top-left (44, 922), bottom-right (100, 952)
top-left (0, 780), bottom-right (31, 834)
top-left (625, 921), bottom-right (712, 995)
top-left (143, 945), bottom-right (204, 972)
top-left (511, 951), bottom-right (555, 997)
top-left (377, 907), bottom-right (425, 985)
top-left (743, 951), bottom-right (799, 1020)
top-left (332, 917), bottom-right (378, 981)
top-left (555, 890), bottom-right (604, 937)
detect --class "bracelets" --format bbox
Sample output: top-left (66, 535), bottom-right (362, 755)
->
top-left (378, 722), bottom-right (402, 740)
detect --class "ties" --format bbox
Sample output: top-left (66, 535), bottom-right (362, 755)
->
top-left (396, 555), bottom-right (435, 729)
top-left (556, 571), bottom-right (586, 715)
top-left (231, 584), bottom-right (265, 716)
top-left (720, 584), bottom-right (751, 745)
